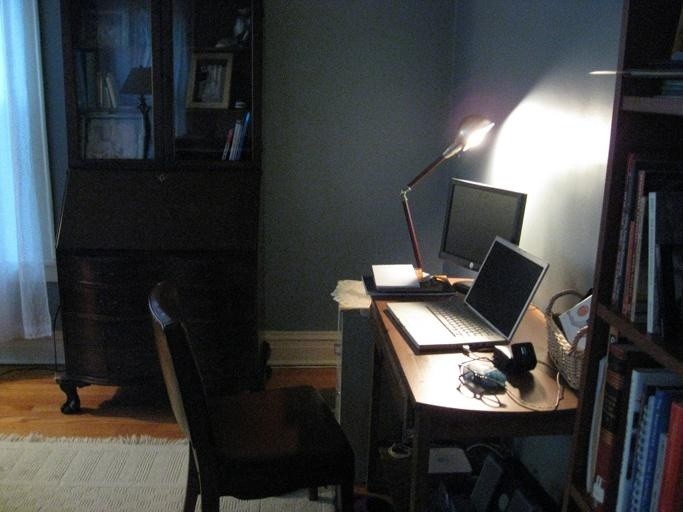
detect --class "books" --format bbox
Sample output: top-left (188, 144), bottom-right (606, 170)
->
top-left (558, 293), bottom-right (592, 350)
top-left (659, 14), bottom-right (682, 98)
top-left (221, 113), bottom-right (251, 160)
top-left (73, 43), bottom-right (120, 112)
top-left (362, 259), bottom-right (457, 296)
top-left (585, 150), bottom-right (682, 511)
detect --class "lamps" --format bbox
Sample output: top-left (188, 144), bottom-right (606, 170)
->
top-left (398, 112), bottom-right (496, 283)
top-left (118, 64), bottom-right (151, 114)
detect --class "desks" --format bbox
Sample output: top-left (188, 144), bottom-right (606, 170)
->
top-left (366, 276), bottom-right (579, 512)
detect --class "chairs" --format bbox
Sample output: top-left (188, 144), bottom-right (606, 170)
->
top-left (141, 276), bottom-right (357, 511)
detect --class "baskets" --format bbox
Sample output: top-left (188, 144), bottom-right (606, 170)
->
top-left (546, 288), bottom-right (593, 390)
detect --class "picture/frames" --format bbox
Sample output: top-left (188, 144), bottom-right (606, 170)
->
top-left (77, 110), bottom-right (148, 162)
top-left (185, 49), bottom-right (233, 110)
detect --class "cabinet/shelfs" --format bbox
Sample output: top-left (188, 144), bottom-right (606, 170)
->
top-left (48, 167), bottom-right (274, 415)
top-left (59, 0), bottom-right (264, 168)
top-left (553, 0), bottom-right (682, 512)
top-left (329, 286), bottom-right (373, 445)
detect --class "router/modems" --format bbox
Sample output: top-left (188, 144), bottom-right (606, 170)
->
top-left (428, 447), bottom-right (473, 475)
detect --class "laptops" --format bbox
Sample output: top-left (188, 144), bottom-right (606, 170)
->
top-left (386, 234), bottom-right (550, 352)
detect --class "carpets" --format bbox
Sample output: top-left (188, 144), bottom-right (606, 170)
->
top-left (0, 429), bottom-right (337, 512)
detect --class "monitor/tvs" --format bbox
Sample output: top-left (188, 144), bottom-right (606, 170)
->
top-left (439, 176), bottom-right (529, 295)
